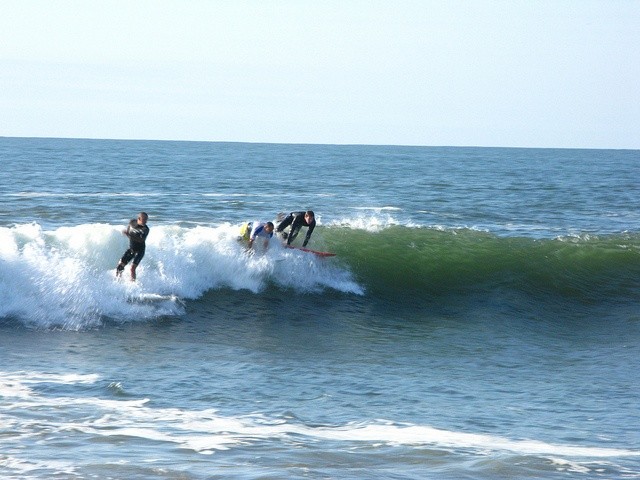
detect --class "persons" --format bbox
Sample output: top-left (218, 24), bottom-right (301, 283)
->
top-left (276, 210), bottom-right (316, 247)
top-left (237, 221), bottom-right (274, 249)
top-left (115, 212), bottom-right (149, 282)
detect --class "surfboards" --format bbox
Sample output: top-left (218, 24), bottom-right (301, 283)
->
top-left (284, 244), bottom-right (336, 256)
top-left (127, 293), bottom-right (177, 303)
